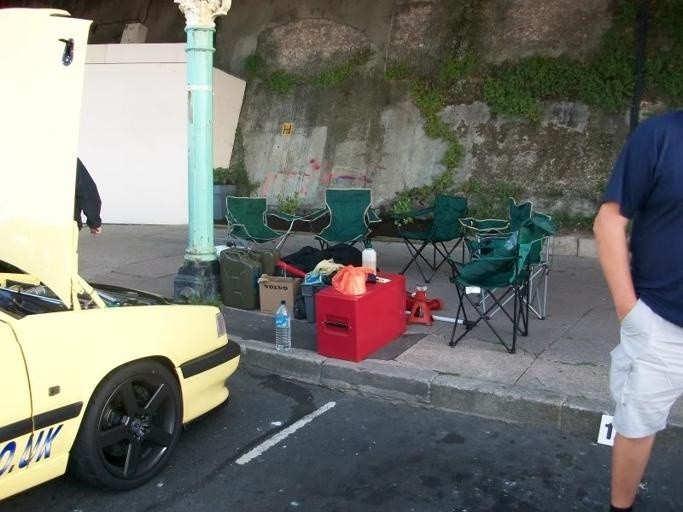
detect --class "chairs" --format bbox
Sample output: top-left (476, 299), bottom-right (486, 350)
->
top-left (224, 194), bottom-right (301, 252)
top-left (297, 186), bottom-right (383, 251)
top-left (442, 229), bottom-right (533, 355)
top-left (457, 194), bottom-right (561, 322)
top-left (392, 192), bottom-right (468, 285)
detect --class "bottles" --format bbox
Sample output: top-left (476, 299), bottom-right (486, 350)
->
top-left (274, 300), bottom-right (291, 350)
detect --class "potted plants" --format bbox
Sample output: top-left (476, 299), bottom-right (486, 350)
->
top-left (210, 163), bottom-right (238, 224)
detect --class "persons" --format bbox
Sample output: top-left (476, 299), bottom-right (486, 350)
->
top-left (74, 156), bottom-right (102, 254)
top-left (593, 104), bottom-right (683, 512)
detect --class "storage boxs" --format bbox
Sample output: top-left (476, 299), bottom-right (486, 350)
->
top-left (299, 282), bottom-right (327, 324)
top-left (256, 272), bottom-right (303, 319)
top-left (313, 269), bottom-right (407, 364)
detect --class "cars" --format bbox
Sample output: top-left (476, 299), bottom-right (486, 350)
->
top-left (0, 7), bottom-right (242, 503)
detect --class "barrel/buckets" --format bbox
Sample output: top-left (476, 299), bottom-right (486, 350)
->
top-left (218, 246), bottom-right (280, 312)
top-left (300, 283), bottom-right (321, 323)
